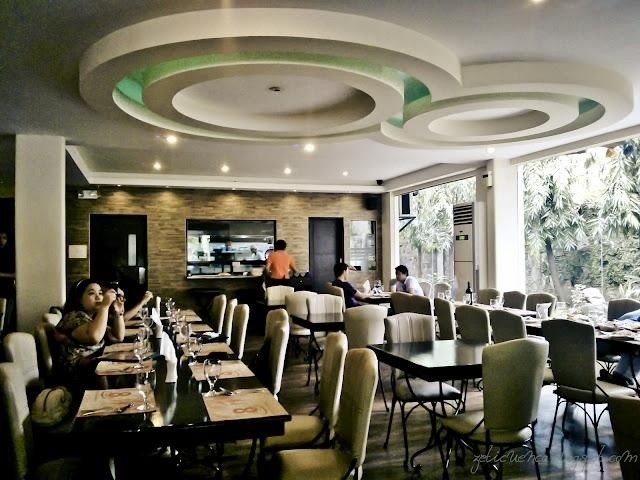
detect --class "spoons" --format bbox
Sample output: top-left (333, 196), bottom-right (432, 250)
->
top-left (219, 385), bottom-right (265, 395)
top-left (83, 403), bottom-right (132, 416)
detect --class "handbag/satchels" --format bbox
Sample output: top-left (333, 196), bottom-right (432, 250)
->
top-left (30, 385), bottom-right (72, 428)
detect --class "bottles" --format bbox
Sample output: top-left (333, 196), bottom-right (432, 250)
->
top-left (465, 280), bottom-right (473, 306)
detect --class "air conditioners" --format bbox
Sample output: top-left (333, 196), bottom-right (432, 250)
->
top-left (399, 190), bottom-right (418, 232)
top-left (451, 201), bottom-right (489, 298)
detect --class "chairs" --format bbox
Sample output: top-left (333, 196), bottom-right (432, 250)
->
top-left (0, 271), bottom-right (640, 480)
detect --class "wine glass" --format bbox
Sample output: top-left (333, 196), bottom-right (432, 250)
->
top-left (133, 307), bottom-right (162, 411)
top-left (166, 297), bottom-right (222, 397)
top-left (368, 280), bottom-right (451, 301)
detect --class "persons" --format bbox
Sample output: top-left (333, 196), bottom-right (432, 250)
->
top-left (221, 236), bottom-right (234, 252)
top-left (266, 239), bottom-right (297, 286)
top-left (333, 263), bottom-right (377, 309)
top-left (103, 283), bottom-right (153, 325)
top-left (394, 264), bottom-right (425, 297)
top-left (249, 245), bottom-right (265, 260)
top-left (0, 230), bottom-right (17, 332)
top-left (57, 278), bottom-right (127, 384)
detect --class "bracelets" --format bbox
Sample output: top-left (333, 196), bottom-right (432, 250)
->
top-left (114, 312), bottom-right (123, 318)
top-left (372, 289), bottom-right (376, 295)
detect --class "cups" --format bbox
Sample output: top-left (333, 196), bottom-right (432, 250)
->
top-left (490, 293), bottom-right (603, 328)
top-left (472, 292), bottom-right (477, 303)
